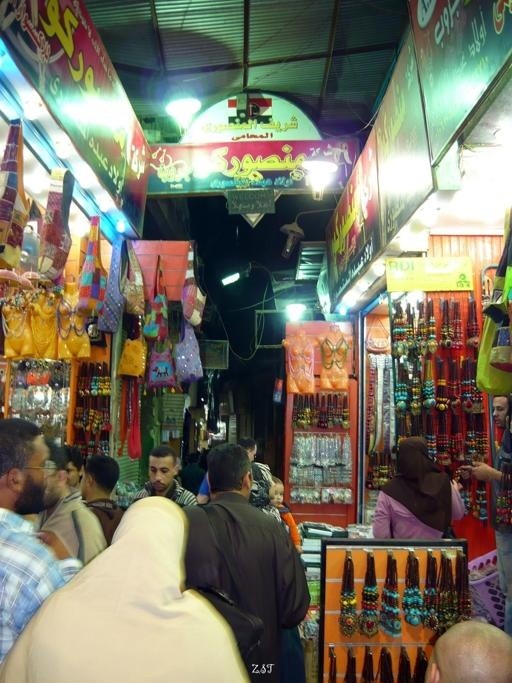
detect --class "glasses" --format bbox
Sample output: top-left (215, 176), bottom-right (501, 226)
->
top-left (25, 460), bottom-right (57, 478)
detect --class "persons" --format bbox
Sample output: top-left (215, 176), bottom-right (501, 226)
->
top-left (372, 436), bottom-right (465, 538)
top-left (425, 620), bottom-right (511, 683)
top-left (1, 419), bottom-right (311, 682)
top-left (459, 396), bottom-right (512, 635)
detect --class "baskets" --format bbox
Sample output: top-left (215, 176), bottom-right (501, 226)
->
top-left (468, 550), bottom-right (504, 628)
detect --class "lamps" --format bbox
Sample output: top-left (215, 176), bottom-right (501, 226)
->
top-left (220, 262), bottom-right (255, 287)
top-left (164, 76), bottom-right (201, 115)
top-left (281, 209), bottom-right (334, 259)
top-left (304, 143), bottom-right (338, 175)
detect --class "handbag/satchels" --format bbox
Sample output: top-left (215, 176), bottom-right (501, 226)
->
top-left (77, 264), bottom-right (146, 335)
top-left (142, 280), bottom-right (207, 388)
top-left (117, 336), bottom-right (147, 377)
top-left (1, 167), bottom-right (31, 270)
top-left (477, 282), bottom-right (511, 398)
top-left (199, 584), bottom-right (266, 657)
top-left (38, 216), bottom-right (71, 281)
